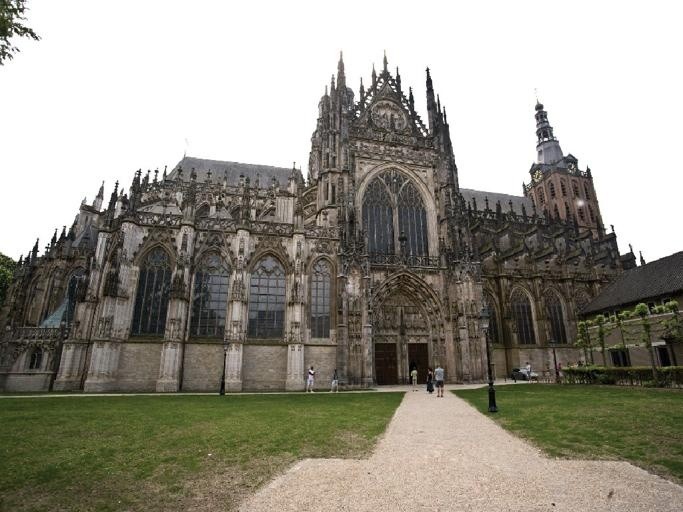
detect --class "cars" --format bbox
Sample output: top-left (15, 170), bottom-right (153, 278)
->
top-left (511, 367), bottom-right (537, 381)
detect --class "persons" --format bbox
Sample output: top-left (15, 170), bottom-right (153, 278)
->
top-left (306, 366), bottom-right (315, 393)
top-left (557, 362), bottom-right (563, 376)
top-left (578, 361), bottom-right (583, 367)
top-left (331, 369), bottom-right (339, 392)
top-left (426, 367), bottom-right (434, 394)
top-left (433, 363), bottom-right (444, 397)
top-left (411, 367), bottom-right (418, 392)
top-left (526, 362), bottom-right (532, 383)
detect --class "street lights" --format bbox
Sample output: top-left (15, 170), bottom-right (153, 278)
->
top-left (217, 338), bottom-right (232, 394)
top-left (475, 306), bottom-right (499, 412)
top-left (549, 338), bottom-right (560, 379)
